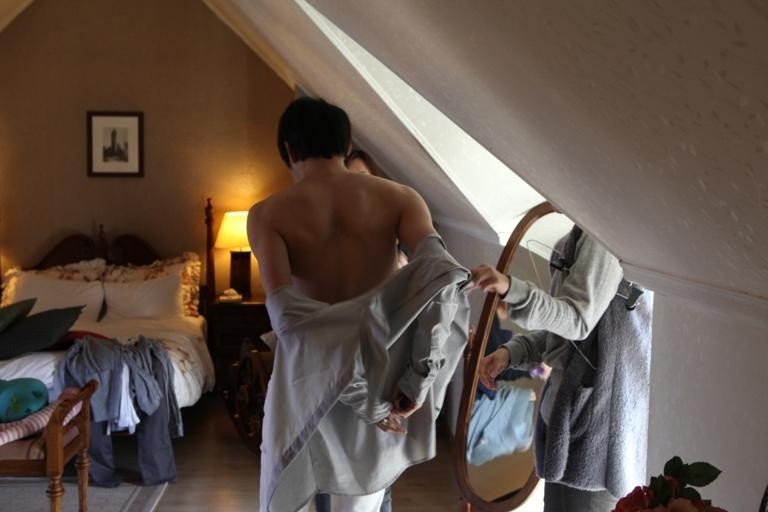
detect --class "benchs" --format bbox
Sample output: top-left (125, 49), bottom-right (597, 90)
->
top-left (0, 379), bottom-right (102, 511)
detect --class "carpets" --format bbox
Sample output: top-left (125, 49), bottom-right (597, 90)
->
top-left (0, 474), bottom-right (169, 510)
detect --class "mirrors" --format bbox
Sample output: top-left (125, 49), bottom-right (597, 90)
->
top-left (455, 201), bottom-right (584, 511)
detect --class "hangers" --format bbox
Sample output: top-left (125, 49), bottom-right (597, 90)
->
top-left (522, 240), bottom-right (633, 368)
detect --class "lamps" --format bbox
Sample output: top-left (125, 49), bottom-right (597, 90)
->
top-left (213, 210), bottom-right (256, 296)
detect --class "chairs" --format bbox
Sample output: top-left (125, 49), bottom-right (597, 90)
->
top-left (465, 377), bottom-right (550, 502)
top-left (223, 340), bottom-right (276, 440)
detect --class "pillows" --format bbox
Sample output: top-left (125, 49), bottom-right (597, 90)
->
top-left (0, 257), bottom-right (108, 308)
top-left (102, 270), bottom-right (186, 319)
top-left (0, 305), bottom-right (88, 358)
top-left (7, 271), bottom-right (103, 323)
top-left (104, 252), bottom-right (203, 318)
top-left (0, 297), bottom-right (38, 334)
top-left (34, 330), bottom-right (109, 352)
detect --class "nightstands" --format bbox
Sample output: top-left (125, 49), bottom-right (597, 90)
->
top-left (208, 293), bottom-right (274, 352)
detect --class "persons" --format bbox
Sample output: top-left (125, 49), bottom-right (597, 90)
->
top-left (246, 97), bottom-right (475, 512)
top-left (470, 221), bottom-right (656, 512)
top-left (475, 301), bottom-right (551, 400)
top-left (343, 149), bottom-right (379, 178)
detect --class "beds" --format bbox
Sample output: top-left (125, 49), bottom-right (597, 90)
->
top-left (0, 223), bottom-right (216, 442)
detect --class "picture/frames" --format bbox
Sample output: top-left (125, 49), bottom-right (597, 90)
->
top-left (86, 110), bottom-right (144, 177)
